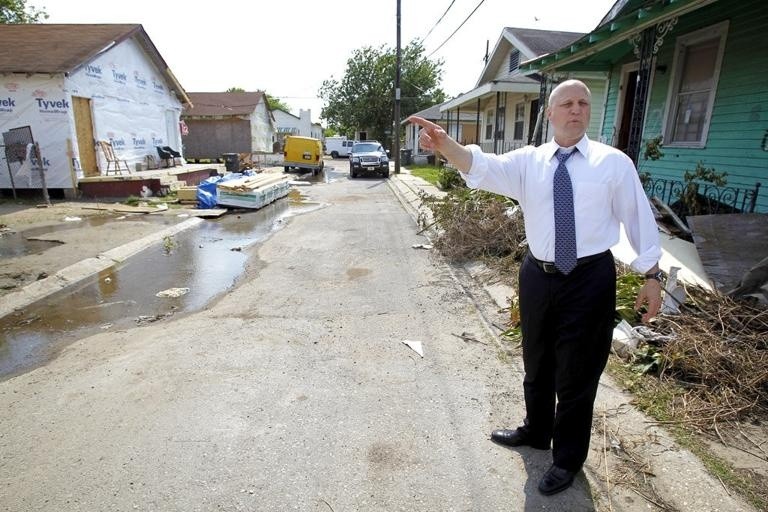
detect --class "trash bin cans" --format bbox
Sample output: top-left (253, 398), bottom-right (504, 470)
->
top-left (401, 148), bottom-right (412, 166)
top-left (222, 153), bottom-right (241, 172)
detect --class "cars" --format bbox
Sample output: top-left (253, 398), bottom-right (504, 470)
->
top-left (350, 143), bottom-right (390, 178)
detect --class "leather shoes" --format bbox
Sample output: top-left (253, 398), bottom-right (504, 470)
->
top-left (491, 425), bottom-right (550, 450)
top-left (538, 463), bottom-right (578, 496)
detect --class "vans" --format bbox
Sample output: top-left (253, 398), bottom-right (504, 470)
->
top-left (283, 136), bottom-right (324, 175)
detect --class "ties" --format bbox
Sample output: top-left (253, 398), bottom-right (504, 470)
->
top-left (548, 152), bottom-right (578, 275)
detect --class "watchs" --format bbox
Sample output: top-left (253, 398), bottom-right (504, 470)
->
top-left (645, 271), bottom-right (664, 282)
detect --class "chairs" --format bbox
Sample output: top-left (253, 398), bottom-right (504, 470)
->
top-left (156, 145), bottom-right (181, 168)
top-left (100, 139), bottom-right (132, 176)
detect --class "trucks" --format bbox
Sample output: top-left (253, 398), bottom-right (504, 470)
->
top-left (327, 139), bottom-right (356, 158)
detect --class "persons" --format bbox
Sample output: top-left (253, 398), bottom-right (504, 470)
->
top-left (410, 79), bottom-right (662, 496)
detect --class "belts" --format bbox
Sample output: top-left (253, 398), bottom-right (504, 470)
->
top-left (527, 248), bottom-right (612, 275)
